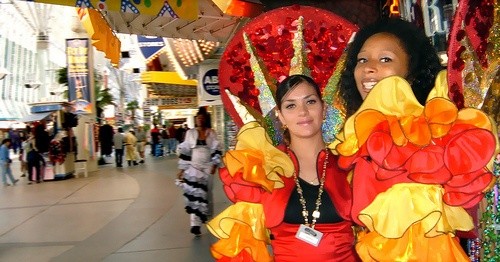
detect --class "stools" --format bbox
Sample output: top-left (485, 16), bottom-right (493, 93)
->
top-left (74, 160), bottom-right (88, 178)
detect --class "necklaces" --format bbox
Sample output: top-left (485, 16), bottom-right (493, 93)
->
top-left (300, 170), bottom-right (318, 184)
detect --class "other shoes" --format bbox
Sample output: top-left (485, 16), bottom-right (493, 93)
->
top-left (189, 227), bottom-right (203, 237)
top-left (12, 179), bottom-right (20, 186)
top-left (3, 183), bottom-right (11, 187)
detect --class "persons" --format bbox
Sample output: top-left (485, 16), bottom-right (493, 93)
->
top-left (0, 120), bottom-right (190, 187)
top-left (328, 15), bottom-right (500, 262)
top-left (204, 74), bottom-right (357, 262)
top-left (174, 106), bottom-right (222, 236)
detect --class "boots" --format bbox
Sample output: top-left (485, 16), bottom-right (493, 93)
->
top-left (128, 160), bottom-right (131, 166)
top-left (132, 160), bottom-right (139, 165)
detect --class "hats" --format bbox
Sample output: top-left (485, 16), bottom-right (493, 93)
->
top-left (129, 128), bottom-right (135, 133)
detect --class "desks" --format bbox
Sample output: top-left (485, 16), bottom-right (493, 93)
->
top-left (55, 155), bottom-right (75, 179)
